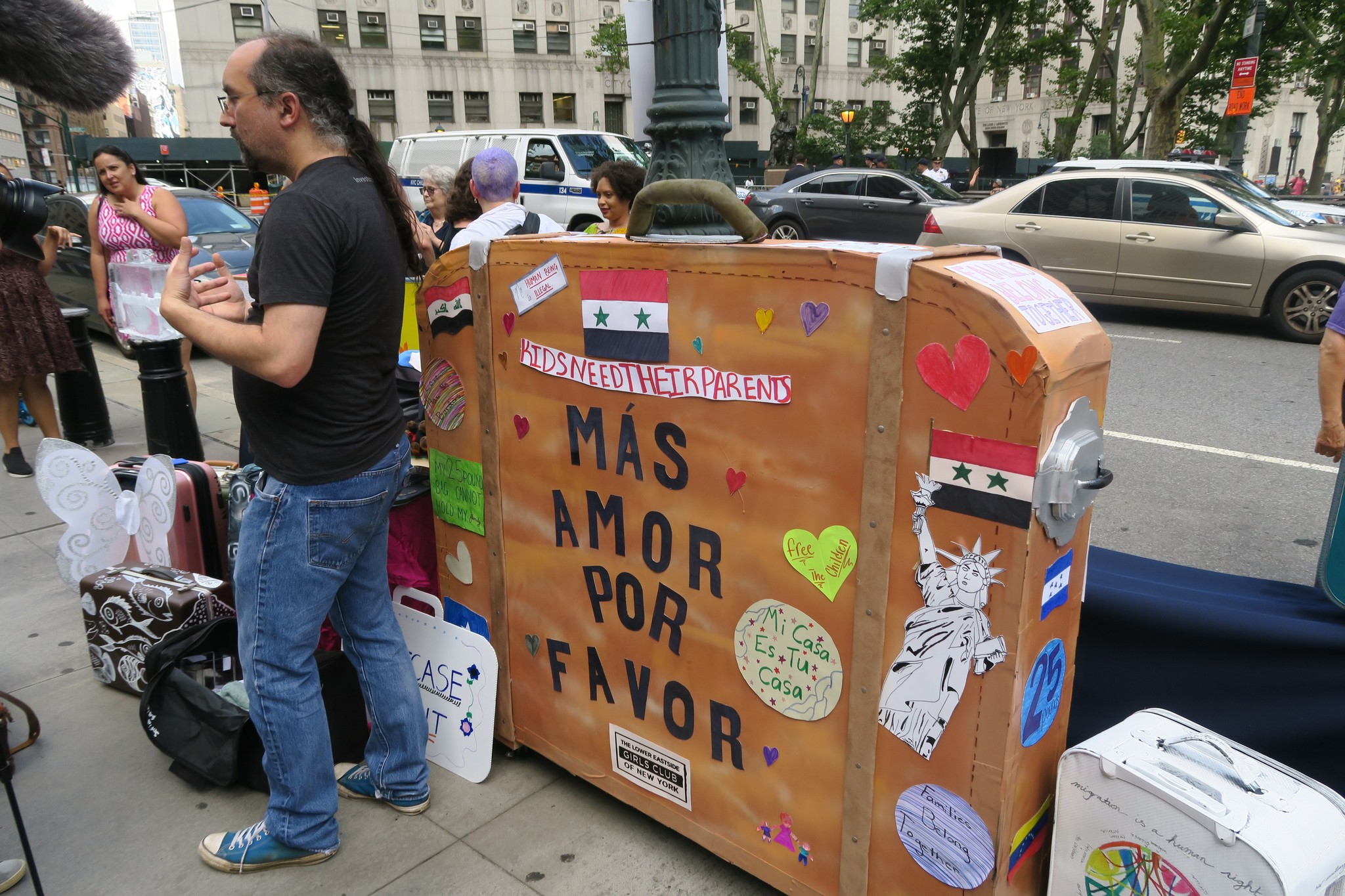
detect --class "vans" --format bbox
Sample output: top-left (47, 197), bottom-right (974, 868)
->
top-left (388, 129), bottom-right (650, 232)
top-left (572, 149), bottom-right (750, 201)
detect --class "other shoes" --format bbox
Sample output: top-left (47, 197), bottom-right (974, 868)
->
top-left (0, 859), bottom-right (26, 894)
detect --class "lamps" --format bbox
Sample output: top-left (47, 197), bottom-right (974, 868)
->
top-left (1027, 93), bottom-right (1035, 99)
top-left (808, 39), bottom-right (815, 46)
top-left (994, 96), bottom-right (1003, 101)
top-left (525, 23), bottom-right (535, 30)
top-left (873, 42), bottom-right (884, 49)
top-left (427, 20), bottom-right (438, 28)
top-left (744, 102), bottom-right (755, 109)
top-left (465, 20), bottom-right (475, 29)
top-left (557, 24), bottom-right (568, 32)
top-left (781, 57), bottom-right (789, 64)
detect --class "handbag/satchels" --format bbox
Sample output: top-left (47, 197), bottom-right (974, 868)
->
top-left (1287, 177), bottom-right (1298, 191)
top-left (140, 617), bottom-right (369, 794)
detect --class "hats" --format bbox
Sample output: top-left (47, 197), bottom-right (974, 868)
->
top-left (993, 179), bottom-right (1002, 187)
top-left (831, 153), bottom-right (844, 160)
top-left (1299, 169), bottom-right (1305, 173)
top-left (916, 158), bottom-right (929, 166)
top-left (864, 154), bottom-right (877, 162)
top-left (931, 156), bottom-right (943, 164)
top-left (875, 156), bottom-right (887, 164)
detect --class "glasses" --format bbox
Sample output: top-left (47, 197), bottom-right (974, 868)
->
top-left (218, 90), bottom-right (300, 114)
top-left (419, 187), bottom-right (447, 195)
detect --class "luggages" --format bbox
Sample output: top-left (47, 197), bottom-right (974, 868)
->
top-left (103, 456), bottom-right (229, 582)
top-left (229, 462), bottom-right (264, 583)
top-left (201, 460), bottom-right (244, 516)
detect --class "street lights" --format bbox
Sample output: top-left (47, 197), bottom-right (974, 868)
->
top-left (839, 104), bottom-right (856, 168)
top-left (792, 64), bottom-right (806, 126)
top-left (1282, 127), bottom-right (1302, 194)
top-left (1037, 110), bottom-right (1050, 158)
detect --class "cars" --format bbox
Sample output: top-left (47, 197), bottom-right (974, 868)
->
top-left (1279, 180), bottom-right (1331, 196)
top-left (916, 169), bottom-right (1345, 344)
top-left (33, 187), bottom-right (259, 359)
top-left (744, 168), bottom-right (981, 245)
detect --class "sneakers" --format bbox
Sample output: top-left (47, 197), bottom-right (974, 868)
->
top-left (197, 820), bottom-right (337, 875)
top-left (2, 447), bottom-right (33, 477)
top-left (334, 762), bottom-right (429, 816)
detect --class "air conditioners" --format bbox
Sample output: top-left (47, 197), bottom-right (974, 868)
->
top-left (814, 102), bottom-right (822, 110)
top-left (367, 16), bottom-right (379, 24)
top-left (240, 6), bottom-right (254, 17)
top-left (853, 104), bottom-right (861, 111)
top-left (326, 12), bottom-right (339, 22)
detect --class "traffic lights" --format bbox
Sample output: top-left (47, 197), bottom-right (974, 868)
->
top-left (1176, 130), bottom-right (1185, 143)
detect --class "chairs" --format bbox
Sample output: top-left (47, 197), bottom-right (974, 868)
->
top-left (1145, 191), bottom-right (1199, 227)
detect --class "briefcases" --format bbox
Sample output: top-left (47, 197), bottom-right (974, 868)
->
top-left (1046, 708), bottom-right (1345, 896)
top-left (79, 561), bottom-right (234, 698)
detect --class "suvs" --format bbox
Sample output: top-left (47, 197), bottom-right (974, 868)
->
top-left (1041, 150), bottom-right (1345, 224)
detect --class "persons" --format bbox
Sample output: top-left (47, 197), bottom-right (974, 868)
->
top-left (157, 31), bottom-right (432, 877)
top-left (865, 153), bottom-right (888, 169)
top-left (87, 144), bottom-right (197, 416)
top-left (939, 167), bottom-right (980, 194)
top-left (782, 153), bottom-right (812, 184)
top-left (990, 178), bottom-right (1009, 196)
top-left (745, 176), bottom-right (754, 186)
top-left (584, 160), bottom-right (648, 234)
top-left (406, 146), bottom-right (564, 279)
top-left (1315, 275), bottom-right (1345, 463)
top-left (1322, 177), bottom-right (1345, 202)
top-left (1287, 169), bottom-right (1307, 195)
top-left (767, 109), bottom-right (797, 167)
top-left (824, 153), bottom-right (846, 170)
top-left (914, 156), bottom-right (949, 193)
top-left (1147, 182), bottom-right (1199, 226)
top-left (0, 857), bottom-right (26, 894)
top-left (0, 164), bottom-right (82, 478)
